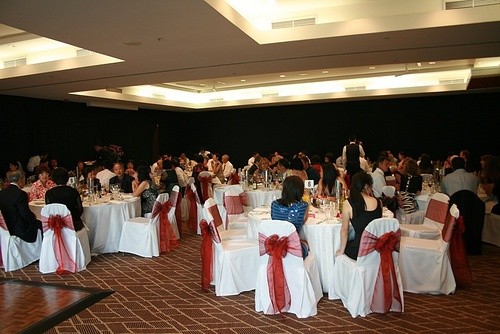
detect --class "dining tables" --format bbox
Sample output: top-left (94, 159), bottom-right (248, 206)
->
top-left (246, 204), bottom-right (394, 292)
top-left (413, 192), bottom-right (487, 211)
top-left (28, 194), bottom-right (141, 256)
top-left (213, 183), bottom-right (282, 209)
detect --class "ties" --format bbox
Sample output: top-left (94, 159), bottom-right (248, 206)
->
top-left (222, 165), bottom-right (226, 172)
top-left (384, 174), bottom-right (387, 186)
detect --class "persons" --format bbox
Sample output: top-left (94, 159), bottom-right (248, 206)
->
top-left (224, 178), bottom-right (249, 214)
top-left (335, 172), bottom-right (383, 260)
top-left (33, 158), bottom-right (48, 181)
top-left (48, 158), bottom-right (58, 179)
top-left (417, 150), bottom-right (493, 185)
top-left (28, 167), bottom-right (58, 202)
top-left (247, 152), bottom-right (343, 198)
top-left (6, 161), bottom-right (25, 188)
top-left (271, 175), bottom-right (309, 260)
top-left (25, 151), bottom-right (48, 179)
top-left (343, 137), bottom-right (366, 186)
top-left (483, 157), bottom-right (500, 215)
top-left (132, 166), bottom-right (159, 217)
top-left (74, 140), bottom-right (137, 192)
top-left (359, 150), bottom-right (422, 191)
top-left (440, 157), bottom-right (479, 198)
top-left (109, 162), bottom-right (136, 193)
top-left (0, 170), bottom-right (43, 243)
top-left (401, 180), bottom-right (422, 214)
top-left (45, 167), bottom-right (84, 232)
top-left (137, 148), bottom-right (233, 198)
top-left (80, 167), bottom-right (101, 194)
top-left (370, 157), bottom-right (401, 218)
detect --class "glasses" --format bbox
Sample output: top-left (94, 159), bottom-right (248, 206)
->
top-left (261, 163), bottom-right (268, 164)
top-left (39, 173), bottom-right (49, 176)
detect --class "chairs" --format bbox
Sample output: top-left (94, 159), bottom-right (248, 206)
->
top-left (39, 204), bottom-right (91, 274)
top-left (0, 210), bottom-right (42, 272)
top-left (119, 172), bottom-right (323, 318)
top-left (329, 193), bottom-right (460, 318)
